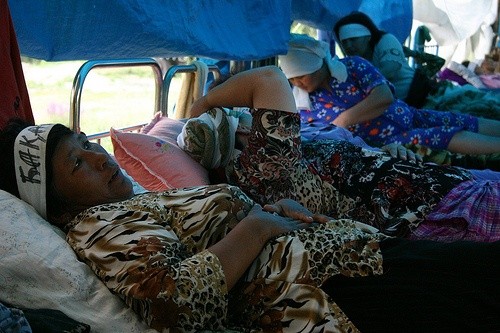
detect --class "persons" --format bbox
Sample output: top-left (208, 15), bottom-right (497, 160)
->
top-left (176, 11), bottom-right (500, 240)
top-left (0, 119), bottom-right (500, 333)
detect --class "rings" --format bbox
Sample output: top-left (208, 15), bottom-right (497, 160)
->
top-left (272, 212), bottom-right (278, 216)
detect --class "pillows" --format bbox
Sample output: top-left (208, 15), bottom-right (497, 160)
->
top-left (0, 190), bottom-right (159, 333)
top-left (111, 110), bottom-right (211, 192)
